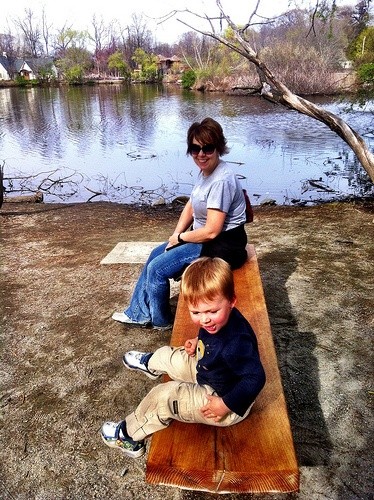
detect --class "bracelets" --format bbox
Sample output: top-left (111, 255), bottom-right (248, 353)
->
top-left (178, 232), bottom-right (183, 242)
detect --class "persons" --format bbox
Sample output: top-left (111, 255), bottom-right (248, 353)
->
top-left (100, 257), bottom-right (266, 458)
top-left (112, 118), bottom-right (247, 330)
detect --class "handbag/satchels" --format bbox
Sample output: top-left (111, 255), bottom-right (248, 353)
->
top-left (242, 189), bottom-right (255, 224)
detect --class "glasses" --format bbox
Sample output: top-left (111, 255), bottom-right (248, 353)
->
top-left (189, 144), bottom-right (216, 155)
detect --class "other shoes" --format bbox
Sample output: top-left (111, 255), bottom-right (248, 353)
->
top-left (112, 312), bottom-right (150, 325)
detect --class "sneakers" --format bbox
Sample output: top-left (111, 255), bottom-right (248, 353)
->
top-left (100, 420), bottom-right (144, 458)
top-left (122, 350), bottom-right (162, 382)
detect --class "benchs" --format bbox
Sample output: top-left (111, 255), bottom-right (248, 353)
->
top-left (146, 244), bottom-right (299, 500)
